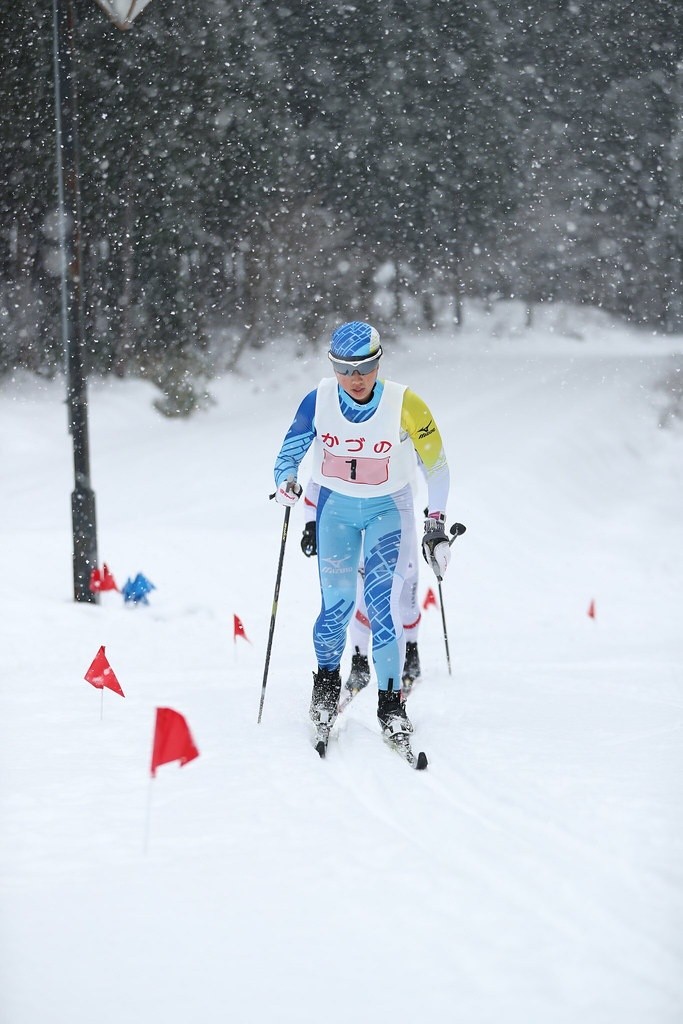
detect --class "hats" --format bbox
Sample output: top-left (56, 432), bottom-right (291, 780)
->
top-left (330, 321), bottom-right (380, 357)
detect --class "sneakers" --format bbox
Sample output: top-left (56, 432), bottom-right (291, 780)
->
top-left (377, 689), bottom-right (413, 736)
top-left (401, 642), bottom-right (421, 680)
top-left (347, 656), bottom-right (370, 689)
top-left (310, 667), bottom-right (342, 726)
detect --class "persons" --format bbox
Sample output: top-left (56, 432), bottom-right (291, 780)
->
top-left (274, 321), bottom-right (450, 742)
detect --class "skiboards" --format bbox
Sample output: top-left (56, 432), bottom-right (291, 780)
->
top-left (337, 688), bottom-right (412, 715)
top-left (313, 730), bottom-right (428, 771)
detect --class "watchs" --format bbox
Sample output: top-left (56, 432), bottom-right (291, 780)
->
top-left (428, 511), bottom-right (446, 524)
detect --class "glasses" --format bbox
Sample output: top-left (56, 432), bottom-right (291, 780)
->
top-left (328, 346), bottom-right (382, 375)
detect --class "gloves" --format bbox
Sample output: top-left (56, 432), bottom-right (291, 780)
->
top-left (424, 540), bottom-right (451, 577)
top-left (269, 481), bottom-right (303, 507)
top-left (301, 521), bottom-right (317, 557)
